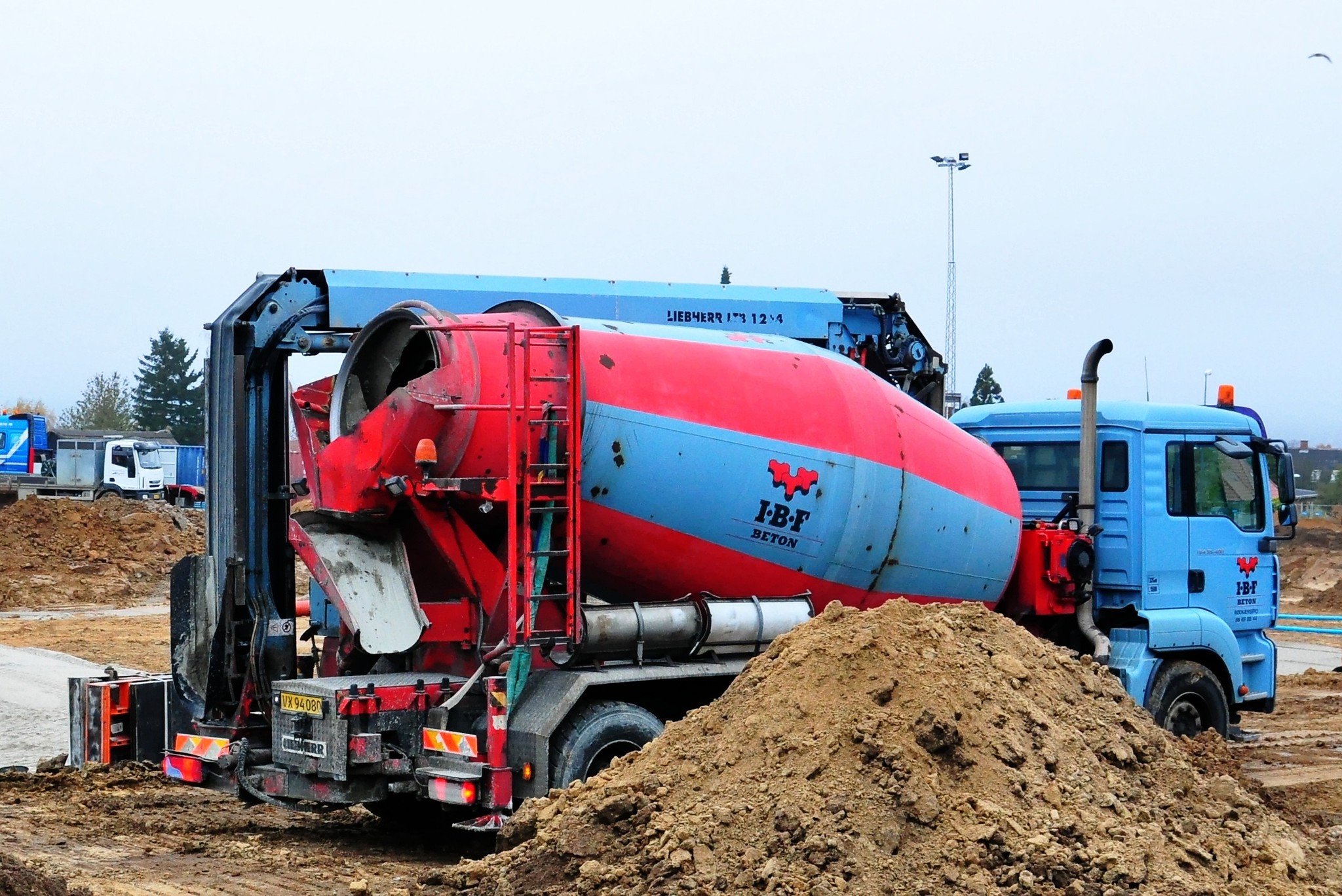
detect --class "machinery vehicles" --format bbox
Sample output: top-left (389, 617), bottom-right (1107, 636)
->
top-left (70, 265), bottom-right (1296, 842)
top-left (0, 409), bottom-right (204, 505)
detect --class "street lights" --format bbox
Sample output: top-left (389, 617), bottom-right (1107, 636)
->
top-left (933, 150), bottom-right (972, 405)
